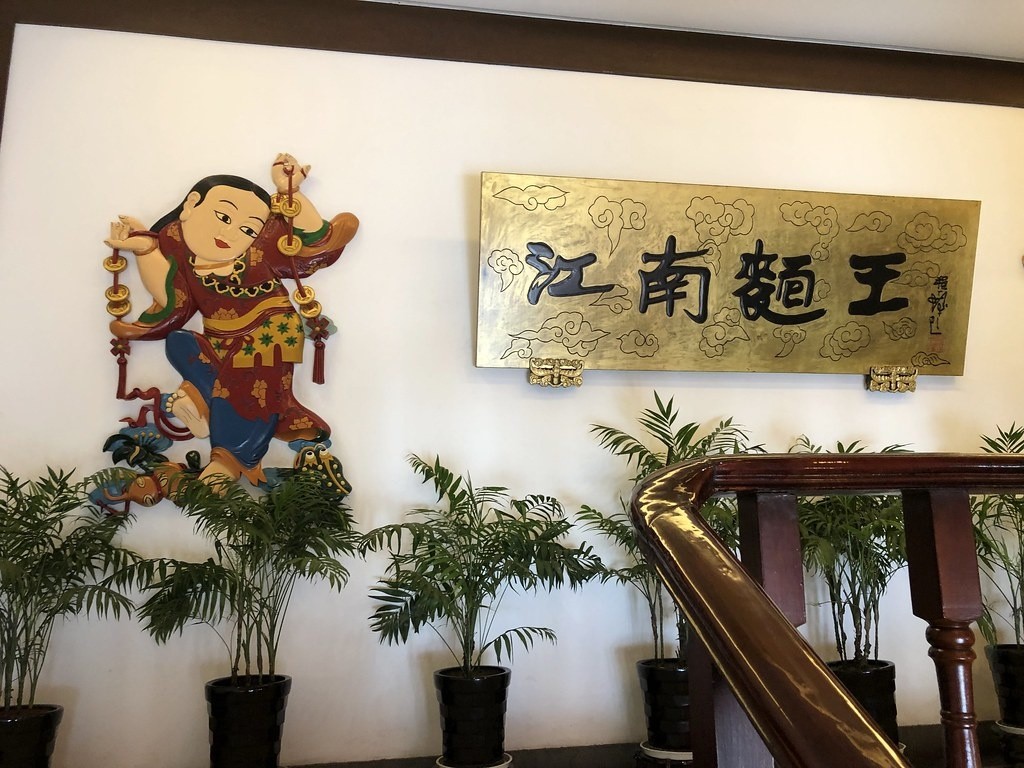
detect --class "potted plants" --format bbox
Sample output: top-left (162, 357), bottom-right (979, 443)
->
top-left (572, 387), bottom-right (768, 759)
top-left (0, 466), bottom-right (181, 768)
top-left (968, 422), bottom-right (1024, 737)
top-left (97, 467), bottom-right (364, 768)
top-left (785, 432), bottom-right (916, 755)
top-left (356, 450), bottom-right (610, 768)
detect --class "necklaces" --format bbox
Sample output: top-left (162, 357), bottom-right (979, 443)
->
top-left (193, 259), bottom-right (234, 270)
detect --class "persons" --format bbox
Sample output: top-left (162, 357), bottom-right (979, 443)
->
top-left (104, 152), bottom-right (360, 500)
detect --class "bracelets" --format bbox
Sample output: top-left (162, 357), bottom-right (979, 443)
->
top-left (278, 186), bottom-right (300, 195)
top-left (134, 240), bottom-right (158, 256)
